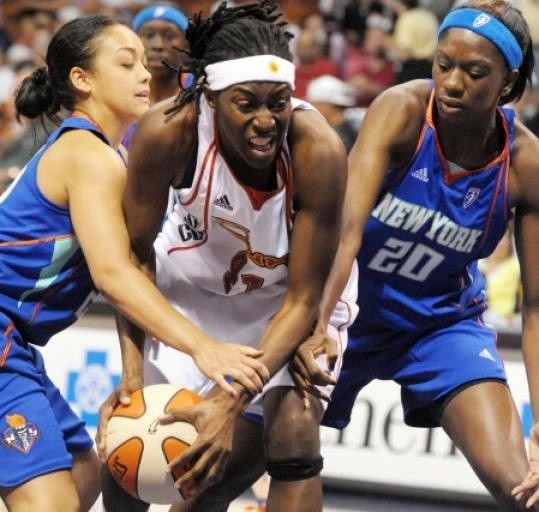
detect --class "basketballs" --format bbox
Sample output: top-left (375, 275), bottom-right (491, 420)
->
top-left (106, 383), bottom-right (208, 504)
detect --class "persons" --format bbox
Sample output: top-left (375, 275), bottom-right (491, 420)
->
top-left (0, 1), bottom-right (194, 193)
top-left (94, 1), bottom-right (360, 512)
top-left (476, 214), bottom-right (524, 319)
top-left (271, 2), bottom-right (441, 151)
top-left (0, 18), bottom-right (270, 512)
top-left (290, 0), bottom-right (539, 512)
